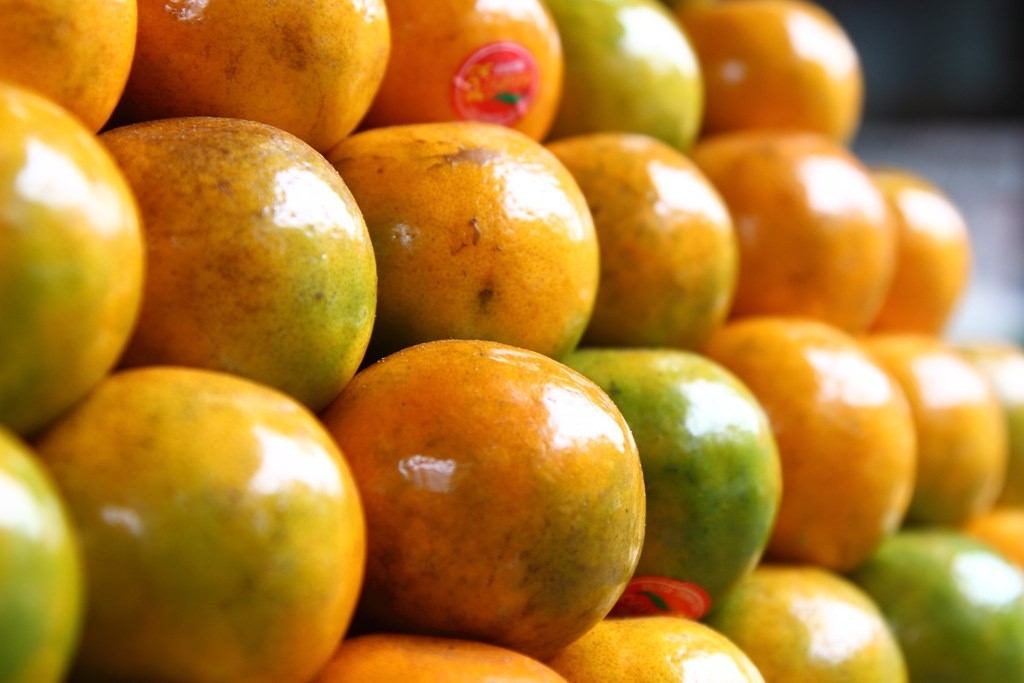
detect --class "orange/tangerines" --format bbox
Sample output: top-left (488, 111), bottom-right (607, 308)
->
top-left (1, 2), bottom-right (1021, 683)
